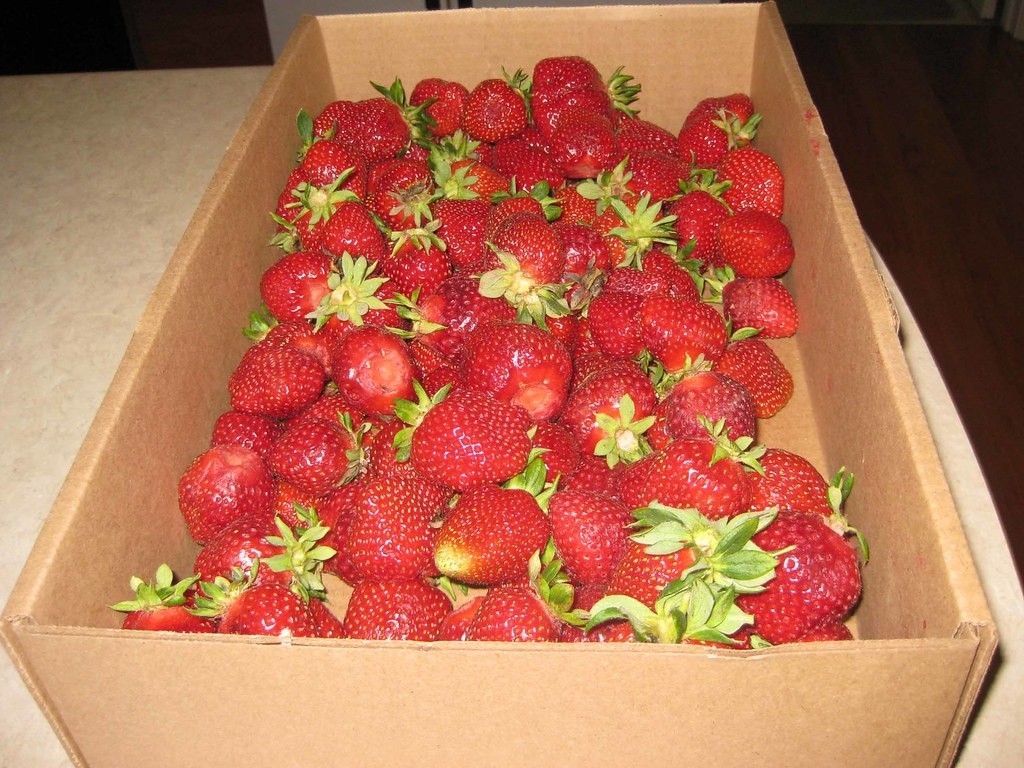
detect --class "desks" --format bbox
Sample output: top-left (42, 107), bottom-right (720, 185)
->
top-left (2, 65), bottom-right (1023, 767)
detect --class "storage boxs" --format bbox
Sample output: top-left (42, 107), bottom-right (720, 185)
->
top-left (1, 1), bottom-right (999, 767)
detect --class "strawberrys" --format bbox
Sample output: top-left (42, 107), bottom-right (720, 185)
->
top-left (110, 56), bottom-right (870, 652)
